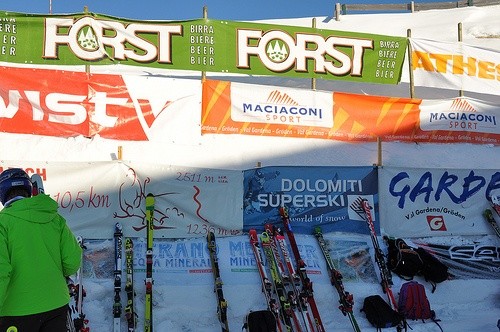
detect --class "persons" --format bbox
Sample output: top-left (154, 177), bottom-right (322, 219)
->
top-left (244, 169), bottom-right (280, 207)
top-left (0, 168), bottom-right (83, 331)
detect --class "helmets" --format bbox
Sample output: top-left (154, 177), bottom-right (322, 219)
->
top-left (0, 168), bottom-right (33, 207)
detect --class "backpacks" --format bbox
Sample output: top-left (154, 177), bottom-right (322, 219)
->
top-left (360, 294), bottom-right (401, 332)
top-left (384, 235), bottom-right (424, 281)
top-left (417, 247), bottom-right (448, 283)
top-left (242, 310), bottom-right (279, 332)
top-left (398, 282), bottom-right (436, 324)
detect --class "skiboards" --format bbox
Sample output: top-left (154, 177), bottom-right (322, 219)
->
top-left (69, 193), bottom-right (407, 332)
top-left (484, 194), bottom-right (500, 237)
